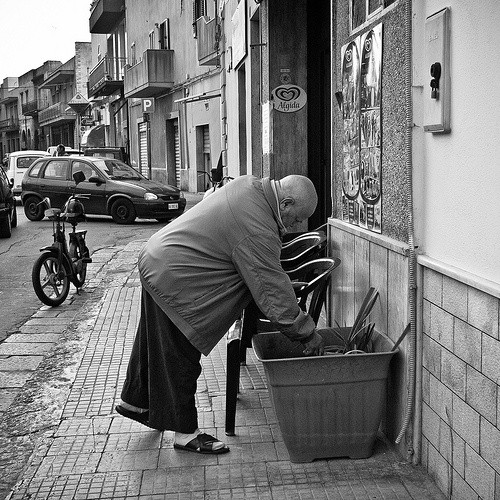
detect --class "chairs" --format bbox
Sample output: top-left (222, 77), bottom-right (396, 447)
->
top-left (280, 231), bottom-right (327, 271)
top-left (225, 256), bottom-right (342, 437)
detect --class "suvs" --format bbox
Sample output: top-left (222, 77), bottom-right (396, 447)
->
top-left (0, 150), bottom-right (50, 198)
top-left (47, 146), bottom-right (125, 168)
top-left (21, 157), bottom-right (187, 224)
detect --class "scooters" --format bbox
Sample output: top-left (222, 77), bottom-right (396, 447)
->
top-left (32, 193), bottom-right (92, 308)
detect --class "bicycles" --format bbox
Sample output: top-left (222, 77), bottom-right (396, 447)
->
top-left (195, 169), bottom-right (236, 199)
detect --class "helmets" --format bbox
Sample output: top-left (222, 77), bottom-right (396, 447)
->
top-left (65, 199), bottom-right (84, 217)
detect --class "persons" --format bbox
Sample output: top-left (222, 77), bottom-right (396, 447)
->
top-left (52, 144), bottom-right (69, 177)
top-left (114, 175), bottom-right (324, 454)
top-left (84, 167), bottom-right (93, 182)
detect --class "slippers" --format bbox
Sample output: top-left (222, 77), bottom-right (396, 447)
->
top-left (115, 405), bottom-right (166, 431)
top-left (174, 433), bottom-right (229, 454)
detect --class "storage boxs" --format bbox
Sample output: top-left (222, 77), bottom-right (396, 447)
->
top-left (251, 326), bottom-right (399, 463)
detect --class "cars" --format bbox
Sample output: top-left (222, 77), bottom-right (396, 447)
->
top-left (0, 166), bottom-right (18, 239)
